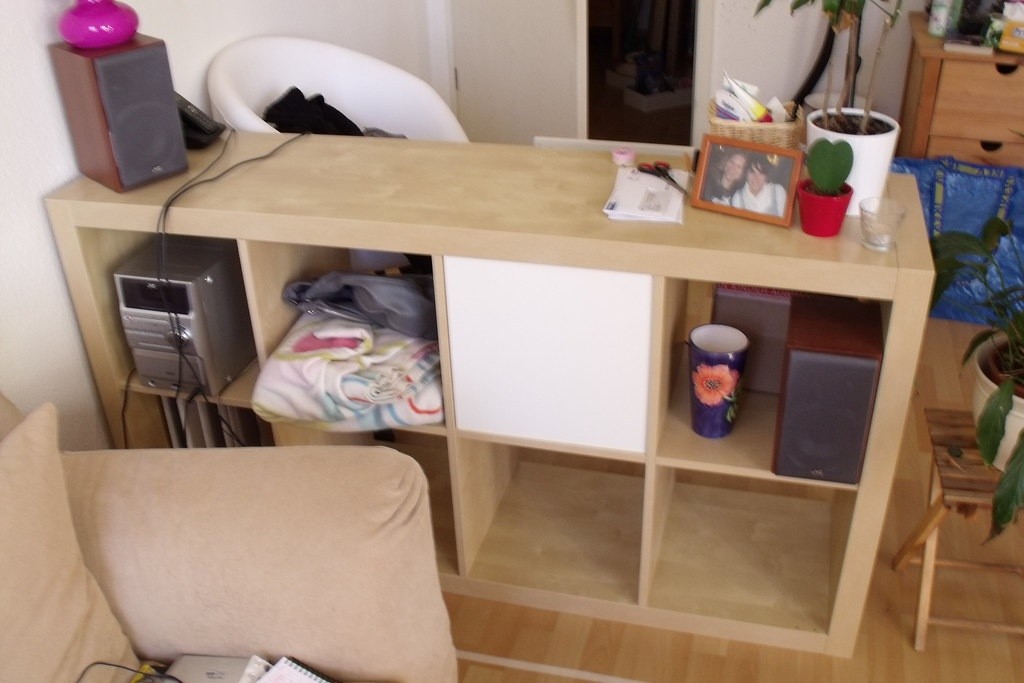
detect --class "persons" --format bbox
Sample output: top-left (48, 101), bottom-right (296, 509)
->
top-left (701, 147), bottom-right (787, 218)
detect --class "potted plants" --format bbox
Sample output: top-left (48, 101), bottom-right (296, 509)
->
top-left (796, 138), bottom-right (853, 237)
top-left (929, 216), bottom-right (1024, 545)
top-left (753, 0), bottom-right (903, 216)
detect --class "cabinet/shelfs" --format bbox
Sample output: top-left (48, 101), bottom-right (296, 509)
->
top-left (44, 131), bottom-right (936, 655)
top-left (895, 12), bottom-right (1024, 167)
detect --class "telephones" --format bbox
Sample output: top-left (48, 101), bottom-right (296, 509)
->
top-left (173, 89), bottom-right (227, 150)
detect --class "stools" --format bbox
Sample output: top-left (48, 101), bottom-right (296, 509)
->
top-left (891, 409), bottom-right (1024, 652)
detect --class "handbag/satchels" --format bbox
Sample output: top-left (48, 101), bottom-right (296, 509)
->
top-left (893, 153), bottom-right (1024, 326)
top-left (283, 270), bottom-right (438, 340)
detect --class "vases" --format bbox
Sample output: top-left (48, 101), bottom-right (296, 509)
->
top-left (688, 324), bottom-right (748, 439)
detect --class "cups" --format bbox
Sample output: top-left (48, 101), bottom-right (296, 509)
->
top-left (689, 325), bottom-right (747, 439)
top-left (927, 0), bottom-right (962, 39)
top-left (858, 196), bottom-right (905, 251)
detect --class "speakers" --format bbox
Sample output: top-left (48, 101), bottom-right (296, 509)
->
top-left (47, 30), bottom-right (189, 193)
top-left (773, 291), bottom-right (885, 482)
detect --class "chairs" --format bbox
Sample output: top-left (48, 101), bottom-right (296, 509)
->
top-left (206, 36), bottom-right (469, 142)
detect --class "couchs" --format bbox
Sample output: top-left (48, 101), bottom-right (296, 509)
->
top-left (0, 392), bottom-right (458, 683)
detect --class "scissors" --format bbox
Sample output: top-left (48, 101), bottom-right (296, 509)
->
top-left (637, 161), bottom-right (690, 196)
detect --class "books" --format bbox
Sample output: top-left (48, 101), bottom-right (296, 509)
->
top-left (254, 657), bottom-right (331, 683)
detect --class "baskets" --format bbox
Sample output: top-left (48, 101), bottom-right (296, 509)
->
top-left (708, 92), bottom-right (804, 152)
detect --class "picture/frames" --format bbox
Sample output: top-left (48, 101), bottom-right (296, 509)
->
top-left (689, 132), bottom-right (803, 228)
